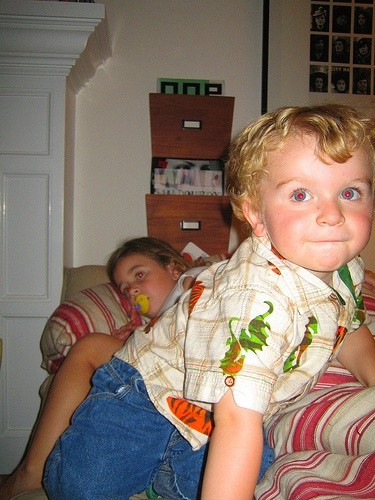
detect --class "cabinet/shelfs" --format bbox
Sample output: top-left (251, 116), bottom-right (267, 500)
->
top-left (146, 94), bottom-right (235, 257)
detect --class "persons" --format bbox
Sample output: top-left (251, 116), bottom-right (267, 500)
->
top-left (353, 72), bottom-right (370, 95)
top-left (333, 12), bottom-right (351, 32)
top-left (42, 104), bottom-right (375, 500)
top-left (332, 38), bottom-right (349, 63)
top-left (310, 72), bottom-right (328, 93)
top-left (353, 38), bottom-right (371, 65)
top-left (354, 12), bottom-right (372, 34)
top-left (0, 237), bottom-right (209, 500)
top-left (312, 7), bottom-right (329, 32)
top-left (310, 37), bottom-right (328, 62)
top-left (332, 71), bottom-right (349, 93)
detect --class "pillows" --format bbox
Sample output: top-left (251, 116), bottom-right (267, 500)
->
top-left (39, 280), bottom-right (141, 375)
